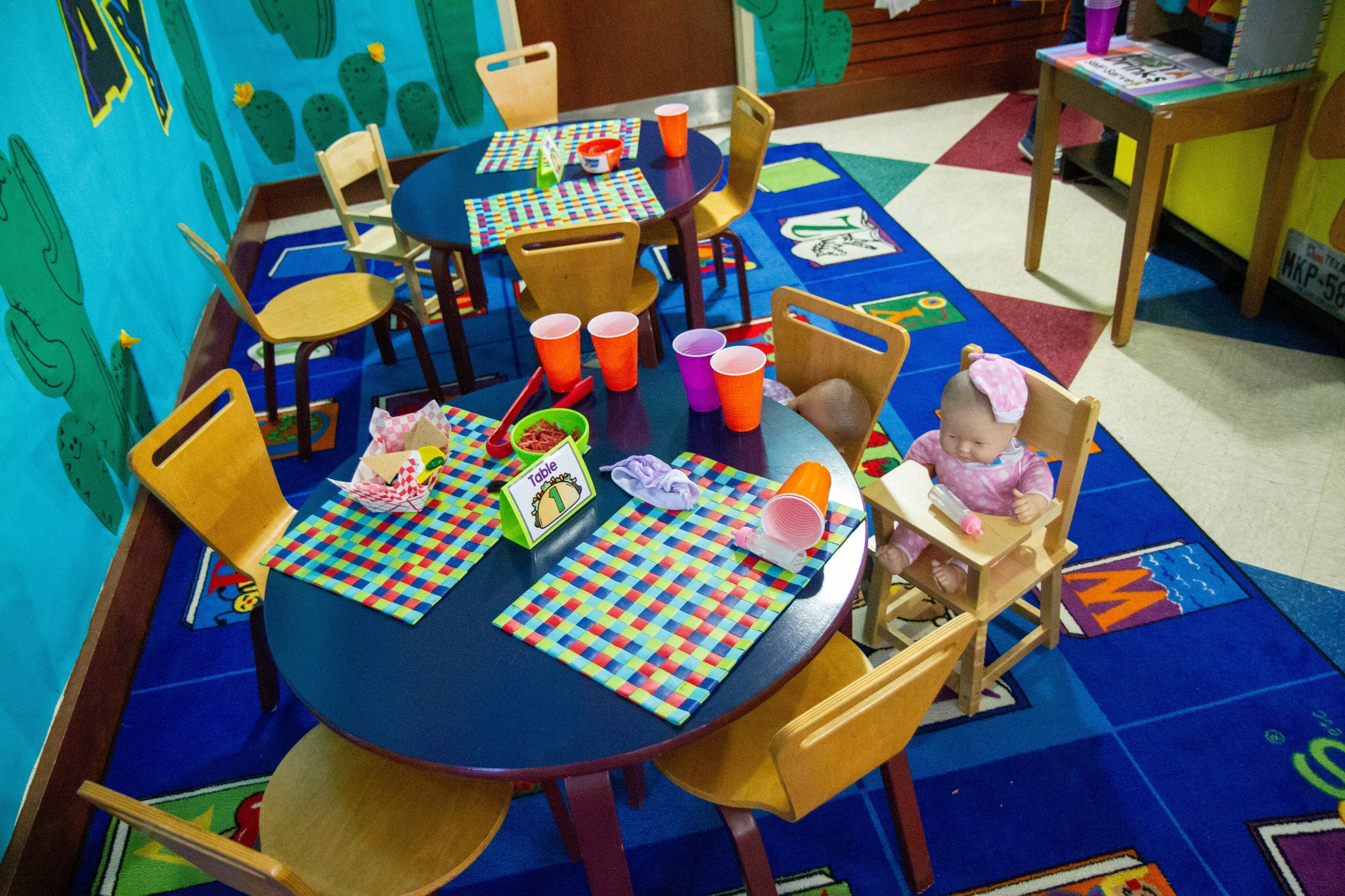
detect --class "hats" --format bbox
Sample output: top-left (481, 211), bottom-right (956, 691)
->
top-left (595, 453), bottom-right (700, 512)
top-left (969, 357), bottom-right (1029, 423)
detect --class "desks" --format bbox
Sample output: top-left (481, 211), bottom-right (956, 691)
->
top-left (266, 364), bottom-right (873, 896)
top-left (1021, 32), bottom-right (1332, 352)
top-left (389, 116), bottom-right (728, 397)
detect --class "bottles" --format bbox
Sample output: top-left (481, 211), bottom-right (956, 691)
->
top-left (927, 484), bottom-right (984, 536)
top-left (731, 526), bottom-right (807, 573)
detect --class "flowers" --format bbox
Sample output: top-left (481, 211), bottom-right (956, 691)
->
top-left (366, 40), bottom-right (387, 65)
top-left (115, 324), bottom-right (146, 354)
top-left (231, 79), bottom-right (254, 112)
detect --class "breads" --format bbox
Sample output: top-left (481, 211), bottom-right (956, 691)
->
top-left (359, 417), bottom-right (450, 485)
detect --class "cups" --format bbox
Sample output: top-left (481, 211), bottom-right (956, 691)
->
top-left (710, 345), bottom-right (767, 432)
top-left (672, 328), bottom-right (727, 412)
top-left (1084, 0), bottom-right (1122, 55)
top-left (762, 461), bottom-right (831, 550)
top-left (529, 313), bottom-right (582, 393)
top-left (654, 103), bottom-right (689, 157)
top-left (587, 311), bottom-right (639, 392)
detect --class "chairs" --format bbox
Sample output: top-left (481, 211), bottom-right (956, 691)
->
top-left (71, 38), bottom-right (1107, 896)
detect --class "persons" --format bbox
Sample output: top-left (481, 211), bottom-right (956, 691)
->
top-left (761, 377), bottom-right (871, 457)
top-left (1017, 0), bottom-right (1132, 176)
top-left (871, 363), bottom-right (1057, 595)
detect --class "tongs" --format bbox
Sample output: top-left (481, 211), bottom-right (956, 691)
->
top-left (485, 366), bottom-right (594, 459)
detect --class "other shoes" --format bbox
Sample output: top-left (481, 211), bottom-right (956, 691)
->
top-left (1018, 135), bottom-right (1060, 172)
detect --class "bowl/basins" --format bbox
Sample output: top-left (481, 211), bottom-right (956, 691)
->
top-left (577, 138), bottom-right (624, 175)
top-left (510, 408), bottom-right (589, 467)
